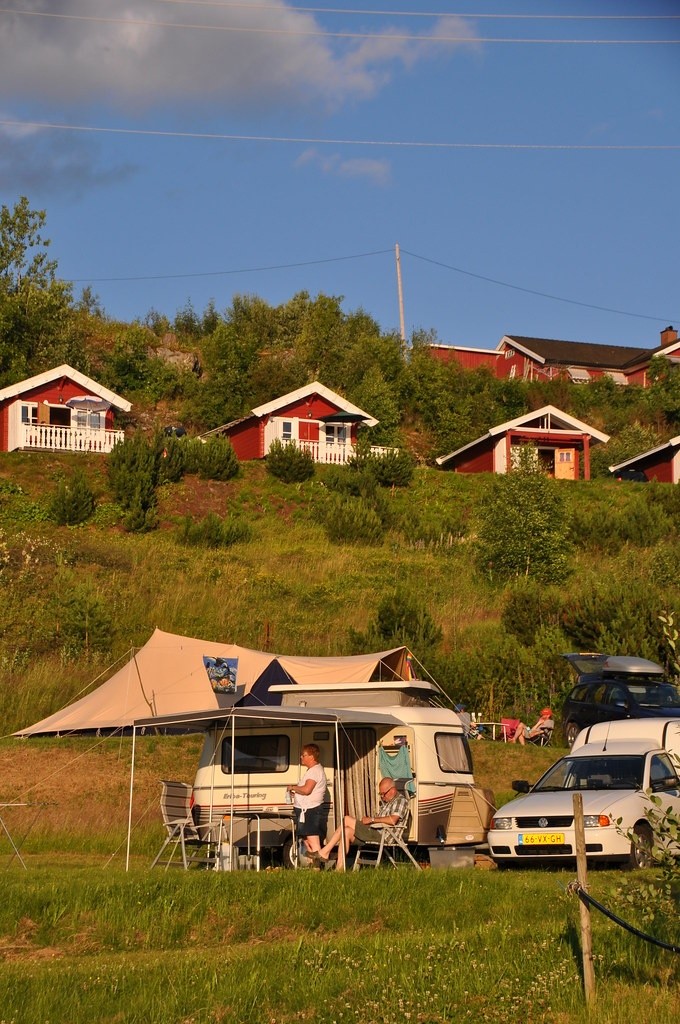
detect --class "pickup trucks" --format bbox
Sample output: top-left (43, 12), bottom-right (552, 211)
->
top-left (488, 715), bottom-right (680, 870)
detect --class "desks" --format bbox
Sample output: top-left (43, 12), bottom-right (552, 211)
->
top-left (234, 813), bottom-right (298, 870)
top-left (469, 722), bottom-right (509, 743)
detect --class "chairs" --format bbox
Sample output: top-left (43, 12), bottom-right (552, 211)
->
top-left (497, 716), bottom-right (519, 743)
top-left (613, 691), bottom-right (627, 704)
top-left (526, 726), bottom-right (554, 746)
top-left (650, 764), bottom-right (665, 781)
top-left (357, 810), bottom-right (421, 867)
top-left (588, 769), bottom-right (612, 786)
top-left (145, 781), bottom-right (226, 872)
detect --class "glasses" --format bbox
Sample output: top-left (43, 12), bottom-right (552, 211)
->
top-left (300, 753), bottom-right (310, 758)
top-left (378, 787), bottom-right (395, 798)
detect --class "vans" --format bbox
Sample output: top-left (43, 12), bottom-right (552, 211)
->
top-left (191, 680), bottom-right (489, 870)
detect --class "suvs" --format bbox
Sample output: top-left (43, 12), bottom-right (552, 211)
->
top-left (558, 652), bottom-right (680, 750)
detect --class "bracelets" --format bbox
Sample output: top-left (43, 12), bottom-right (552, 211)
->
top-left (372, 818), bottom-right (374, 824)
top-left (292, 786), bottom-right (293, 790)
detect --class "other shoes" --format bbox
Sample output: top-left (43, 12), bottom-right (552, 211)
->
top-left (306, 861), bottom-right (321, 871)
top-left (507, 737), bottom-right (516, 744)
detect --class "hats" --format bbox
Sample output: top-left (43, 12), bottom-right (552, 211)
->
top-left (541, 708), bottom-right (553, 716)
top-left (454, 704), bottom-right (466, 711)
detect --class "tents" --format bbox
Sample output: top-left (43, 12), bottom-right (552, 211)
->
top-left (12, 624), bottom-right (486, 740)
top-left (126, 706), bottom-right (409, 873)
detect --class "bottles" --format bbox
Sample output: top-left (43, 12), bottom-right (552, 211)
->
top-left (525, 726), bottom-right (532, 738)
top-left (471, 712), bottom-right (475, 723)
top-left (286, 789), bottom-right (291, 804)
top-left (478, 713), bottom-right (482, 723)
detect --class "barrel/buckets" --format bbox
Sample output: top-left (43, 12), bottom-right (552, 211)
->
top-left (213, 842), bottom-right (239, 871)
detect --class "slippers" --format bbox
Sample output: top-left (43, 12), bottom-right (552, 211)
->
top-left (305, 850), bottom-right (328, 862)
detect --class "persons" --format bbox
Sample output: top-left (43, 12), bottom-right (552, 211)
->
top-left (287, 744), bottom-right (327, 871)
top-left (508, 708), bottom-right (555, 745)
top-left (304, 777), bottom-right (409, 874)
top-left (455, 704), bottom-right (482, 740)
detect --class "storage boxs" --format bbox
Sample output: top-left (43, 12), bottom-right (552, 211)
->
top-left (428, 846), bottom-right (476, 868)
